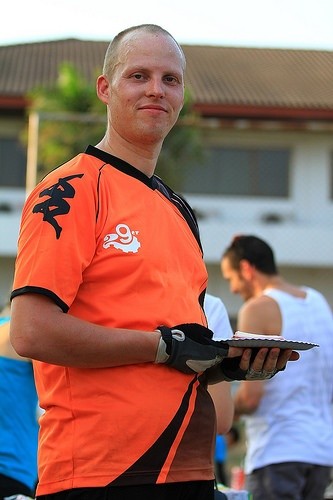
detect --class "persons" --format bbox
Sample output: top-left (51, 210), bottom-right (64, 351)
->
top-left (9, 22), bottom-right (302, 500)
top-left (0, 300), bottom-right (40, 500)
top-left (204, 293), bottom-right (248, 487)
top-left (220, 233), bottom-right (332, 500)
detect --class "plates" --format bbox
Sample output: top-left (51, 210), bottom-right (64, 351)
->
top-left (210, 335), bottom-right (321, 352)
